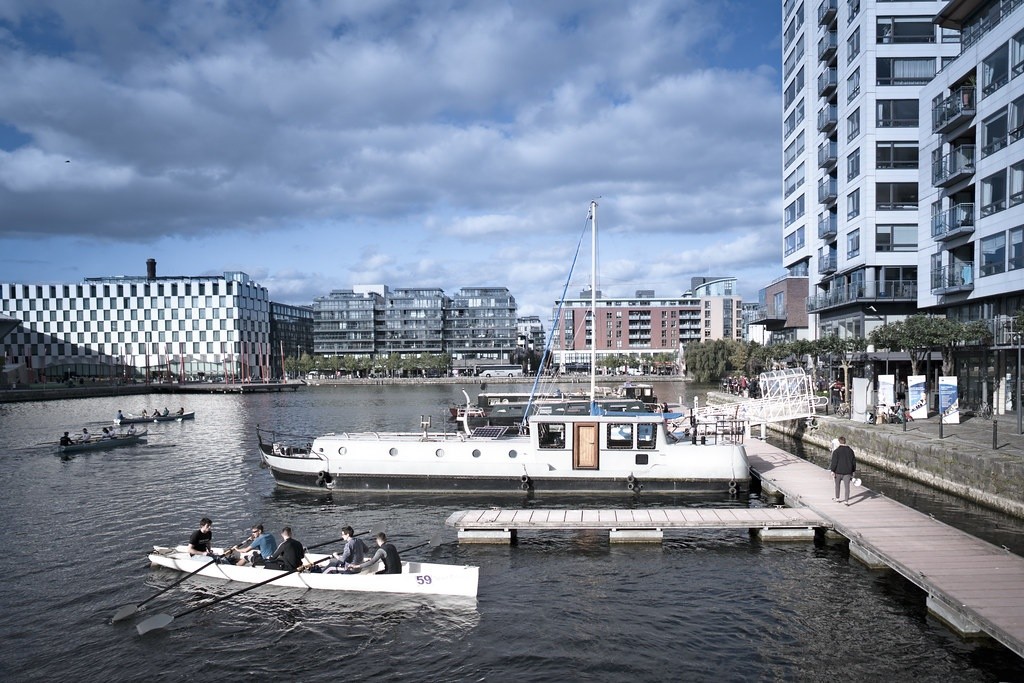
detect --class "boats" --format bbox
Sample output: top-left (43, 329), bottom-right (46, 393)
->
top-left (50, 428), bottom-right (147, 453)
top-left (147, 544), bottom-right (480, 597)
top-left (448, 385), bottom-right (661, 426)
top-left (253, 202), bottom-right (755, 498)
top-left (112, 412), bottom-right (195, 426)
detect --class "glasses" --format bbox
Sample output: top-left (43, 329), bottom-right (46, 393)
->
top-left (342, 533), bottom-right (345, 536)
top-left (251, 530), bottom-right (259, 533)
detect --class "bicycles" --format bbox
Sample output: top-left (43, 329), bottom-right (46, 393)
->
top-left (833, 401), bottom-right (851, 419)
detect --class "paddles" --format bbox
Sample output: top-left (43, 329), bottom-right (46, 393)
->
top-left (397, 534), bottom-right (442, 553)
top-left (111, 535), bottom-right (253, 620)
top-left (304, 529), bottom-right (372, 549)
top-left (135, 553), bottom-right (340, 635)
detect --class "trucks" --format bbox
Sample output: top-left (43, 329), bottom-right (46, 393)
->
top-left (627, 368), bottom-right (645, 376)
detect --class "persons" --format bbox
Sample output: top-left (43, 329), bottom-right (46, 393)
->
top-left (154, 409), bottom-right (161, 416)
top-left (60, 431), bottom-right (76, 446)
top-left (897, 379), bottom-right (907, 409)
top-left (117, 410), bottom-right (125, 420)
top-left (187, 518), bottom-right (217, 562)
top-left (109, 426), bottom-right (115, 440)
top-left (831, 437), bottom-right (856, 506)
top-left (352, 532), bottom-right (402, 575)
top-left (322, 526), bottom-right (369, 574)
top-left (888, 405), bottom-right (914, 424)
top-left (162, 407), bottom-right (169, 417)
top-left (720, 371), bottom-right (845, 414)
top-left (102, 428), bottom-right (112, 440)
top-left (127, 423), bottom-right (137, 434)
top-left (266, 526), bottom-right (304, 571)
top-left (78, 428), bottom-right (91, 443)
top-left (142, 410), bottom-right (147, 416)
top-left (233, 524), bottom-right (277, 566)
top-left (177, 407), bottom-right (184, 415)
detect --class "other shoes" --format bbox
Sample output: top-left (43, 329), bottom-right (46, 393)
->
top-left (832, 498), bottom-right (841, 503)
top-left (843, 501), bottom-right (849, 506)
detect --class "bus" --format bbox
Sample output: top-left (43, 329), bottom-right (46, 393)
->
top-left (308, 371), bottom-right (318, 379)
top-left (477, 365), bottom-right (524, 377)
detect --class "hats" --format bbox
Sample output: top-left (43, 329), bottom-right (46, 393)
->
top-left (729, 377), bottom-right (732, 379)
top-left (734, 376), bottom-right (737, 378)
